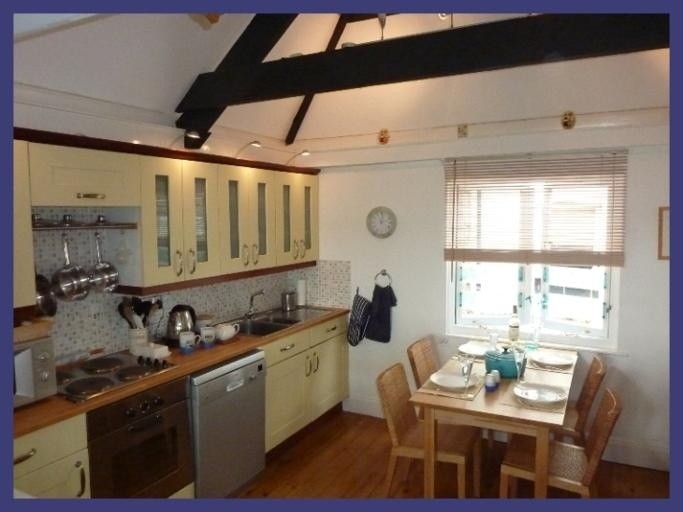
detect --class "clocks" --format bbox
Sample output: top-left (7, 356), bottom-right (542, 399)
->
top-left (366, 206), bottom-right (396, 238)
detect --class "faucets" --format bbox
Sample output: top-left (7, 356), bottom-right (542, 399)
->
top-left (244, 288), bottom-right (265, 321)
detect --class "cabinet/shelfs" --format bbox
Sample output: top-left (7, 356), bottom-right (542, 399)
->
top-left (257, 315), bottom-right (348, 455)
top-left (11, 414), bottom-right (92, 501)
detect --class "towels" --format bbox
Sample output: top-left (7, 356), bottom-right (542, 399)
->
top-left (345, 283), bottom-right (398, 347)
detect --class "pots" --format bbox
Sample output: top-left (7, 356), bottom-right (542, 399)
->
top-left (35, 228), bottom-right (121, 321)
top-left (484, 346), bottom-right (527, 378)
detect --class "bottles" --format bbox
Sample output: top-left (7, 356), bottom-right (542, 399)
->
top-left (295, 275), bottom-right (306, 308)
top-left (509, 306), bottom-right (520, 342)
top-left (485, 369), bottom-right (500, 391)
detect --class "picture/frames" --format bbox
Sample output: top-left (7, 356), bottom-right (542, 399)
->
top-left (657, 206), bottom-right (671, 261)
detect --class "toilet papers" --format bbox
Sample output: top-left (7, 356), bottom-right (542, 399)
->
top-left (296, 278), bottom-right (307, 306)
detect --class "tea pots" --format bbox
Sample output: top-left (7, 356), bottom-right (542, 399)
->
top-left (213, 323), bottom-right (240, 341)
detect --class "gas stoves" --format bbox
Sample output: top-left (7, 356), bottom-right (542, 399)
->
top-left (53, 350), bottom-right (178, 400)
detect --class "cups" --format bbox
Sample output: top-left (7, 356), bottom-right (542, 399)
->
top-left (281, 290), bottom-right (296, 311)
top-left (179, 327), bottom-right (216, 354)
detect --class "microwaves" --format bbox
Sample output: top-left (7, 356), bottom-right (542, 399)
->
top-left (13, 333), bottom-right (57, 411)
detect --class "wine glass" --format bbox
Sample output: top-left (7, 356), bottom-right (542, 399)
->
top-left (460, 355), bottom-right (473, 398)
top-left (512, 347), bottom-right (525, 383)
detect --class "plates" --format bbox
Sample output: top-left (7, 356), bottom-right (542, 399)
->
top-left (513, 381), bottom-right (569, 406)
top-left (155, 190), bottom-right (207, 266)
top-left (530, 348), bottom-right (576, 366)
top-left (430, 370), bottom-right (479, 389)
top-left (459, 342), bottom-right (495, 355)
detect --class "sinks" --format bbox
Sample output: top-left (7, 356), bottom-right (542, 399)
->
top-left (259, 316), bottom-right (301, 326)
top-left (232, 319), bottom-right (291, 339)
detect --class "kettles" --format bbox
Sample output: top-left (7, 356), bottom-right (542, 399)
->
top-left (169, 302), bottom-right (196, 342)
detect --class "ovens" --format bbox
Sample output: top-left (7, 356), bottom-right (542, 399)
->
top-left (78, 374), bottom-right (195, 498)
